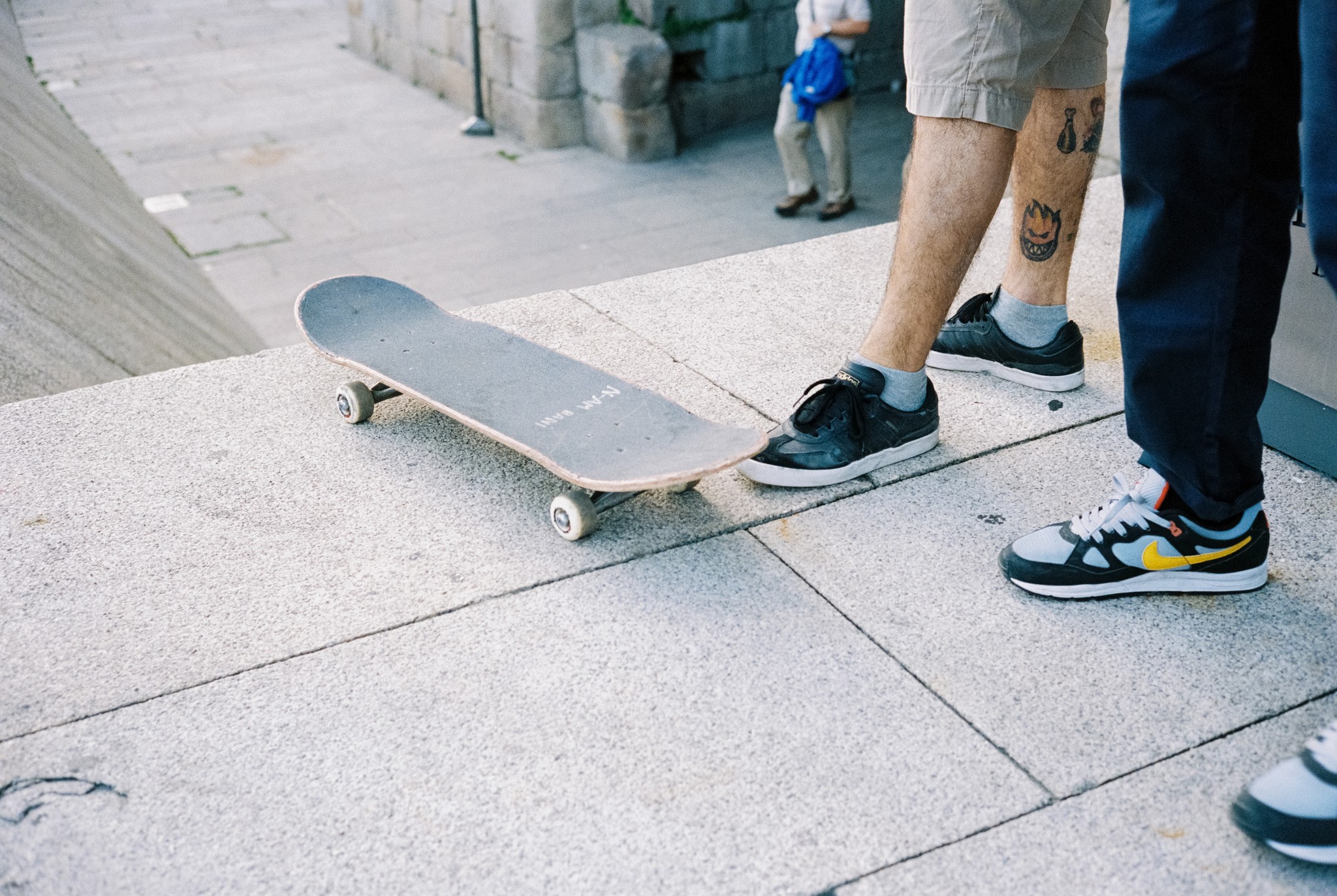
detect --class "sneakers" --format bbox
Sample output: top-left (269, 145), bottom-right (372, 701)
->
top-left (997, 468), bottom-right (1270, 605)
top-left (925, 283), bottom-right (1085, 393)
top-left (1232, 718), bottom-right (1337, 867)
top-left (733, 353), bottom-right (940, 488)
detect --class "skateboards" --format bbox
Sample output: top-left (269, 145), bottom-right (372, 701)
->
top-left (292, 273), bottom-right (770, 541)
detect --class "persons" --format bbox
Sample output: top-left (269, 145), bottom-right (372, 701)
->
top-left (738, 0), bottom-right (1111, 487)
top-left (773, 0), bottom-right (871, 220)
top-left (998, 0), bottom-right (1337, 865)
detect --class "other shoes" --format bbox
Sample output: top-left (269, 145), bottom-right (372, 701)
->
top-left (817, 197), bottom-right (855, 221)
top-left (775, 186), bottom-right (819, 217)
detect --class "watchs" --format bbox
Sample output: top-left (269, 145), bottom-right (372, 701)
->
top-left (822, 21), bottom-right (832, 34)
